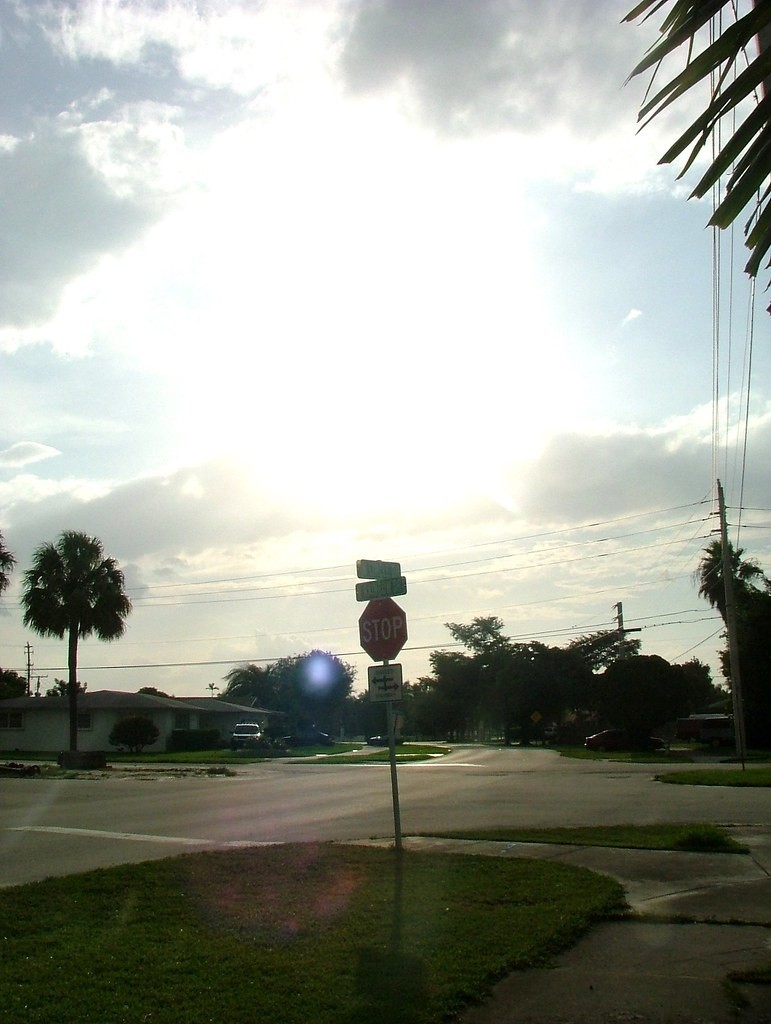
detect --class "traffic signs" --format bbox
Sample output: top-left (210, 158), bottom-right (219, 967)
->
top-left (355, 559), bottom-right (407, 602)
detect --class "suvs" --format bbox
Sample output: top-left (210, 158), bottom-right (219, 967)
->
top-left (231, 723), bottom-right (261, 750)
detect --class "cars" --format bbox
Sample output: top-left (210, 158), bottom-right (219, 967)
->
top-left (584, 729), bottom-right (668, 753)
top-left (365, 736), bottom-right (404, 746)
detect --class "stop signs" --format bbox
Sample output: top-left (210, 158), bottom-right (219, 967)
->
top-left (358, 599), bottom-right (408, 661)
